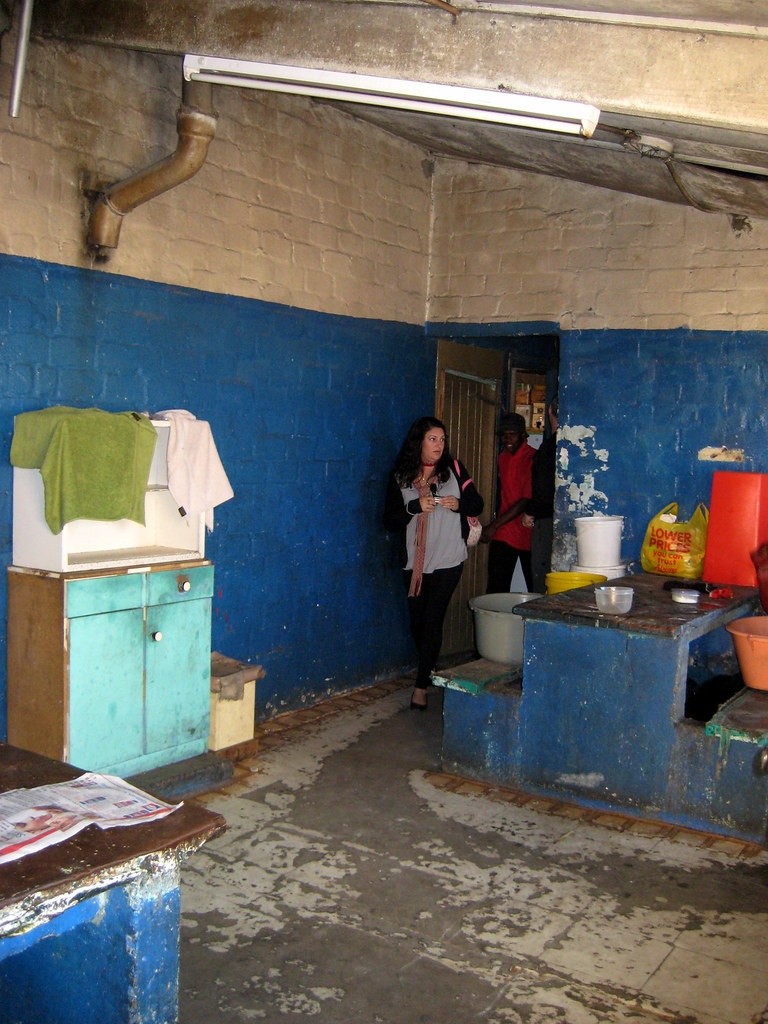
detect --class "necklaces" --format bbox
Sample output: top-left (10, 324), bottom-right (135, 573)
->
top-left (422, 462), bottom-right (435, 481)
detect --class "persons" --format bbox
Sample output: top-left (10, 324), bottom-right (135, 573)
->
top-left (481, 412), bottom-right (537, 594)
top-left (532, 396), bottom-right (559, 593)
top-left (381, 416), bottom-right (484, 713)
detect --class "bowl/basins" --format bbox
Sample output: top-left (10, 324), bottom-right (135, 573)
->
top-left (594, 586), bottom-right (634, 614)
top-left (672, 588), bottom-right (700, 604)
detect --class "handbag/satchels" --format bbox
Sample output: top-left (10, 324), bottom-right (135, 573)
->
top-left (467, 516), bottom-right (482, 546)
top-left (640, 502), bottom-right (710, 579)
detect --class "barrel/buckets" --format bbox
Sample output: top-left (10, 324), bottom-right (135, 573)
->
top-left (569, 561), bottom-right (626, 581)
top-left (573, 516), bottom-right (624, 567)
top-left (545, 572), bottom-right (607, 595)
top-left (468, 592), bottom-right (544, 665)
top-left (725, 616), bottom-right (768, 691)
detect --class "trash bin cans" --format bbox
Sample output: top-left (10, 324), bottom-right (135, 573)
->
top-left (211, 651), bottom-right (268, 749)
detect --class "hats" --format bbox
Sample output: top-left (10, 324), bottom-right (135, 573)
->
top-left (499, 413), bottom-right (529, 438)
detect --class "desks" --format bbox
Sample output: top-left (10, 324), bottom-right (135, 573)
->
top-left (1, 742), bottom-right (226, 1024)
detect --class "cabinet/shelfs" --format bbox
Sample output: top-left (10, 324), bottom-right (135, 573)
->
top-left (5, 557), bottom-right (217, 781)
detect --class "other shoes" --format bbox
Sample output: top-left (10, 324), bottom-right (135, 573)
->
top-left (410, 691), bottom-right (428, 711)
top-left (427, 664), bottom-right (437, 687)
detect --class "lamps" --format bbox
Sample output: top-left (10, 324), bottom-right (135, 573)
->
top-left (182, 54), bottom-right (601, 139)
